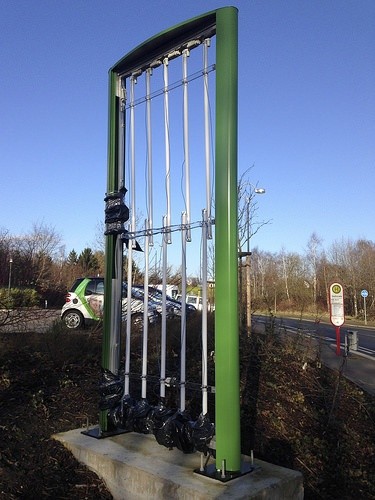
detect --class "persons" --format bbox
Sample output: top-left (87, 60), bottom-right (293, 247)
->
top-left (86, 295), bottom-right (103, 317)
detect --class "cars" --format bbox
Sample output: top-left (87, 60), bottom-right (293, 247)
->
top-left (61, 277), bottom-right (215, 337)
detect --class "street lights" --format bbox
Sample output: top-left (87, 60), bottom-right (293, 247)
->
top-left (7, 253), bottom-right (14, 297)
